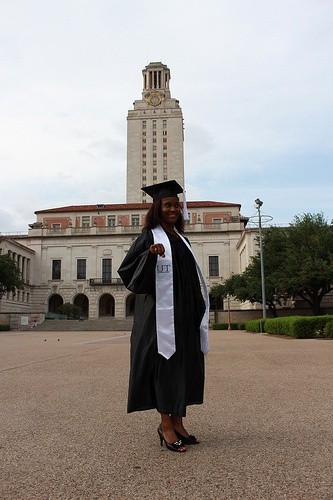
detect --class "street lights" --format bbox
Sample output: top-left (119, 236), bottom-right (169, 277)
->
top-left (253, 199), bottom-right (266, 319)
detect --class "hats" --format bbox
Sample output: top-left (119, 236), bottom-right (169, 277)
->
top-left (141, 180), bottom-right (189, 221)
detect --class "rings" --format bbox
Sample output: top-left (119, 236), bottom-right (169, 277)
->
top-left (154, 247), bottom-right (157, 251)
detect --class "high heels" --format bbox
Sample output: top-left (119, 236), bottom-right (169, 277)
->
top-left (174, 428), bottom-right (200, 444)
top-left (157, 424), bottom-right (186, 452)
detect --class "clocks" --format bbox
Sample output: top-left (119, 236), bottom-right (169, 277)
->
top-left (147, 93), bottom-right (161, 106)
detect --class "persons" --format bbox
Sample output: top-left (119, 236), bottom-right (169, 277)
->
top-left (30, 320), bottom-right (36, 328)
top-left (116, 180), bottom-right (209, 453)
top-left (78, 313), bottom-right (85, 321)
